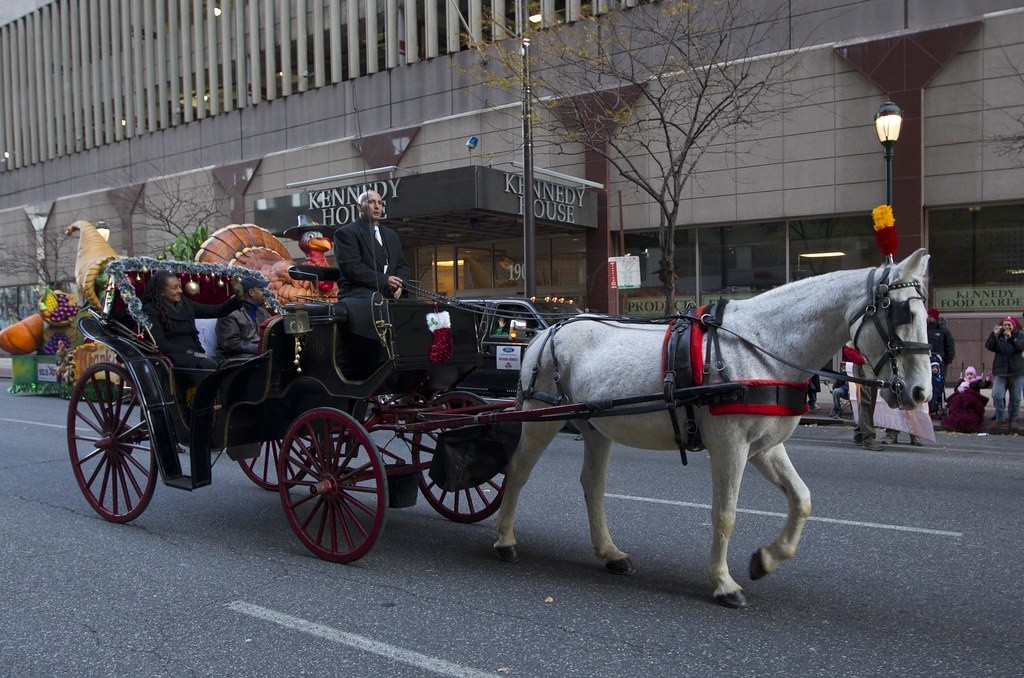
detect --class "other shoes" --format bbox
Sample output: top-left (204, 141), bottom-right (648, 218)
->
top-left (882, 432), bottom-right (898, 444)
top-left (1009, 421), bottom-right (1018, 430)
top-left (911, 436), bottom-right (924, 446)
top-left (990, 420), bottom-right (1006, 430)
top-left (852, 438), bottom-right (864, 445)
top-left (833, 412), bottom-right (840, 419)
top-left (863, 438), bottom-right (885, 450)
top-left (812, 404), bottom-right (821, 410)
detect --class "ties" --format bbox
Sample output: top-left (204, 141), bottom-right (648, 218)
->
top-left (373, 225), bottom-right (382, 246)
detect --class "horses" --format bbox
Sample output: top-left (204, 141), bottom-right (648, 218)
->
top-left (487, 247), bottom-right (937, 607)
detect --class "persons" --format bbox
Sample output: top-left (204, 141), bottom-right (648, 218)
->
top-left (927, 352), bottom-right (945, 416)
top-left (335, 189), bottom-right (409, 368)
top-left (842, 338), bottom-right (887, 450)
top-left (217, 277), bottom-right (273, 362)
top-left (881, 427), bottom-right (924, 446)
top-left (831, 380), bottom-right (849, 419)
top-left (927, 308), bottom-right (955, 373)
top-left (940, 365), bottom-right (992, 432)
top-left (986, 316), bottom-right (1024, 432)
top-left (141, 269), bottom-right (244, 382)
top-left (808, 374), bottom-right (821, 410)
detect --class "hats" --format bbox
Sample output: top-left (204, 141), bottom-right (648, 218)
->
top-left (240, 275), bottom-right (266, 289)
top-left (927, 307), bottom-right (939, 321)
top-left (1003, 316), bottom-right (1019, 328)
top-left (965, 366), bottom-right (977, 376)
top-left (930, 355), bottom-right (940, 367)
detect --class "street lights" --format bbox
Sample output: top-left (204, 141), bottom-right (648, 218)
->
top-left (95, 216), bottom-right (111, 244)
top-left (872, 90), bottom-right (905, 266)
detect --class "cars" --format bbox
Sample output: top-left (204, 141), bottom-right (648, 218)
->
top-left (450, 290), bottom-right (588, 438)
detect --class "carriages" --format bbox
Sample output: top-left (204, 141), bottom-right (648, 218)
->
top-left (65, 205), bottom-right (935, 610)
